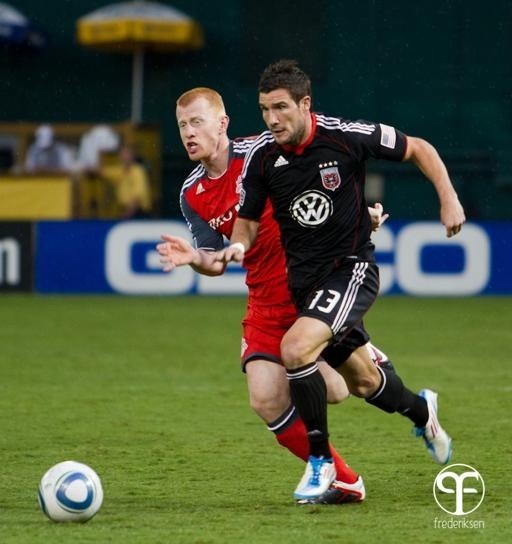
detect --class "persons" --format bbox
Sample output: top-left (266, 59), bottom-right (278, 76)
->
top-left (155, 87), bottom-right (395, 505)
top-left (210, 59), bottom-right (467, 501)
top-left (0, 123), bottom-right (153, 220)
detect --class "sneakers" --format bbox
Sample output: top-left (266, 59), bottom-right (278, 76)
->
top-left (416, 388), bottom-right (452, 465)
top-left (298, 475), bottom-right (366, 506)
top-left (294, 456), bottom-right (336, 500)
top-left (366, 342), bottom-right (394, 373)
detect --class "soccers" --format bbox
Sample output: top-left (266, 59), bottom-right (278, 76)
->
top-left (38, 459), bottom-right (103, 522)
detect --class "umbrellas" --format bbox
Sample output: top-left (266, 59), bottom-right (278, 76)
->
top-left (76, 0), bottom-right (208, 123)
